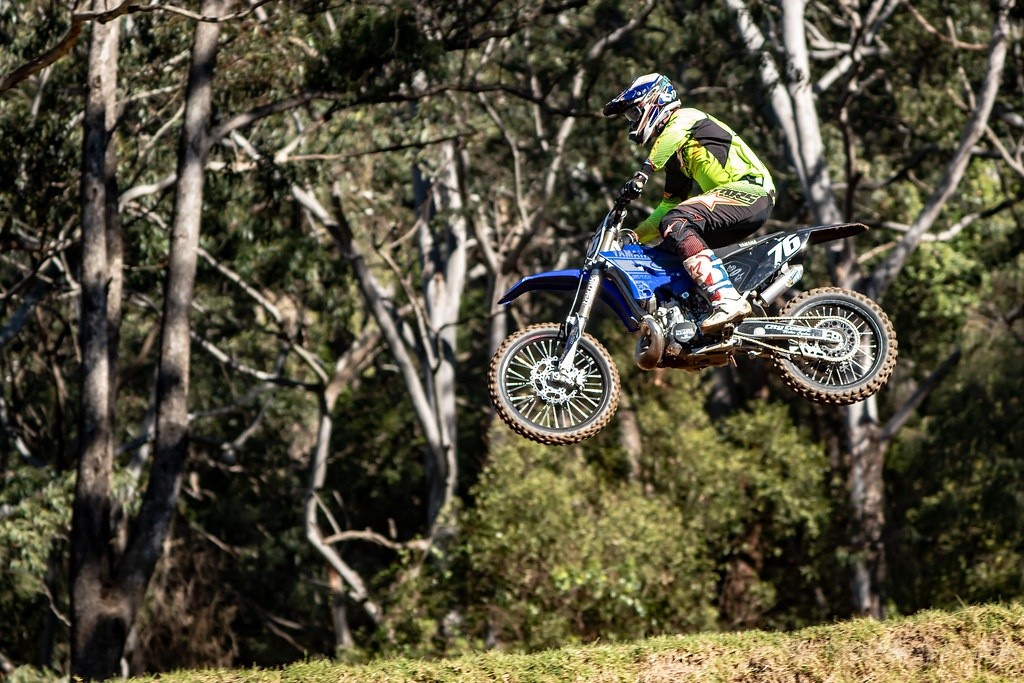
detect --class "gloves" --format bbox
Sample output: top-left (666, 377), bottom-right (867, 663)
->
top-left (620, 173), bottom-right (648, 200)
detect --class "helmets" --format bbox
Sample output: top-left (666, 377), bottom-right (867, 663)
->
top-left (602, 72), bottom-right (681, 147)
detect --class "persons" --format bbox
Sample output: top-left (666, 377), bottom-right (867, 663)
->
top-left (602, 73), bottom-right (778, 334)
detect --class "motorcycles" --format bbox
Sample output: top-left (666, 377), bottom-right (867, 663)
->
top-left (486, 175), bottom-right (898, 445)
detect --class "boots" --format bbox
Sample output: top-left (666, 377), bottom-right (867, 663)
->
top-left (683, 247), bottom-right (754, 330)
